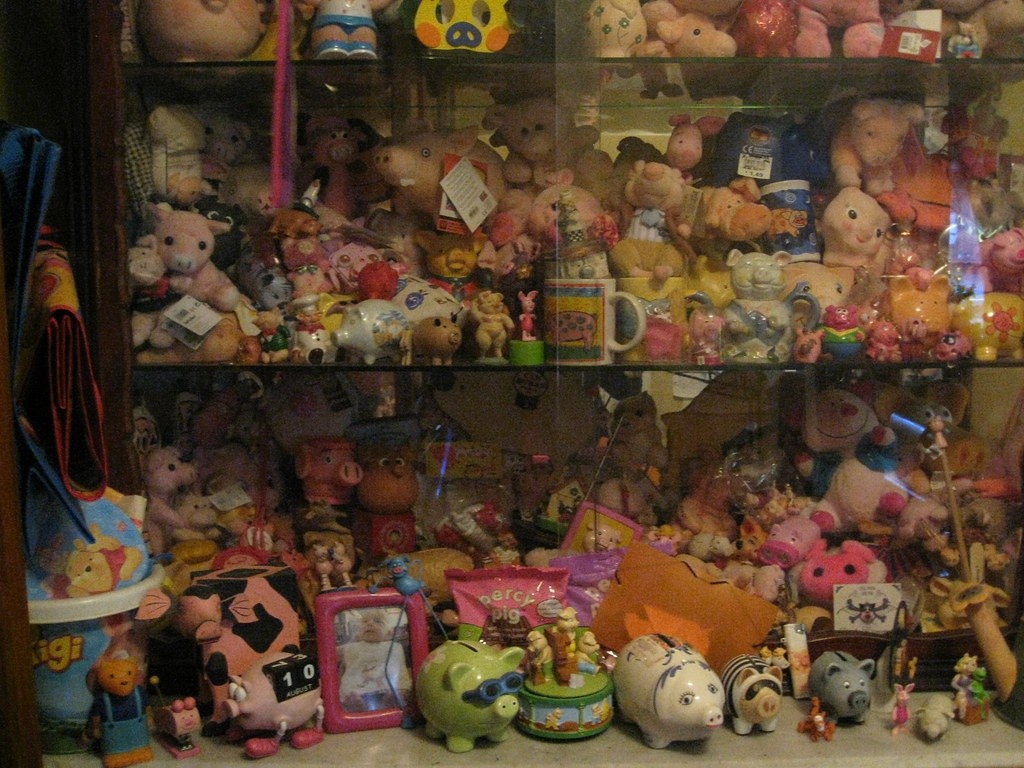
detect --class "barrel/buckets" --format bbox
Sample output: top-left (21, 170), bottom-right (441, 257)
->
top-left (27, 563), bottom-right (162, 754)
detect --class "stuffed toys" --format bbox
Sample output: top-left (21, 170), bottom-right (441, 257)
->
top-left (114, 0), bottom-right (1024, 365)
top-left (84, 368), bottom-right (1023, 637)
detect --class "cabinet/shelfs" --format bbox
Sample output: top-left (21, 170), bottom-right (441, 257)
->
top-left (87, 0), bottom-right (1024, 692)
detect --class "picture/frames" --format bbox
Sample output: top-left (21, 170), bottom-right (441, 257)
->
top-left (315, 586), bottom-right (429, 733)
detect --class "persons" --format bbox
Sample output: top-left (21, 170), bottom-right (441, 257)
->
top-left (334, 608), bottom-right (410, 712)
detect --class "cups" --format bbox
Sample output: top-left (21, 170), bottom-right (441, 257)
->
top-left (545, 278), bottom-right (646, 364)
